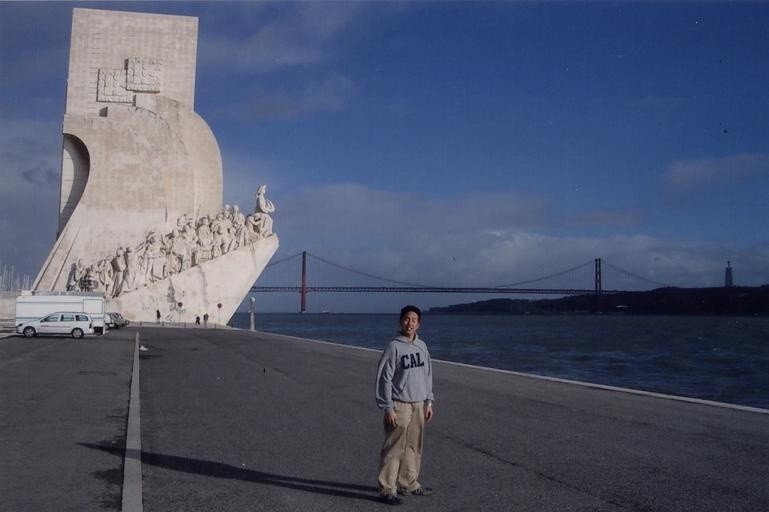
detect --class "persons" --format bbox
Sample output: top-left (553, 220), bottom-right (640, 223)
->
top-left (155, 309), bottom-right (161, 324)
top-left (203, 312), bottom-right (209, 327)
top-left (195, 315), bottom-right (201, 325)
top-left (374, 304), bottom-right (437, 506)
top-left (65, 184), bottom-right (276, 296)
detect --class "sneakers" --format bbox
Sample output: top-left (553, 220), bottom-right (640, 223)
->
top-left (380, 496), bottom-right (404, 505)
top-left (412, 486), bottom-right (435, 497)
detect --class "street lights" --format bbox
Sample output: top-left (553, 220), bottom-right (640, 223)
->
top-left (248, 296), bottom-right (258, 330)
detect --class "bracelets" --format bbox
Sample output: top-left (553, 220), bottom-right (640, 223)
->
top-left (427, 403), bottom-right (432, 406)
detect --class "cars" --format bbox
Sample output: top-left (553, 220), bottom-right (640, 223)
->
top-left (12, 311), bottom-right (130, 340)
